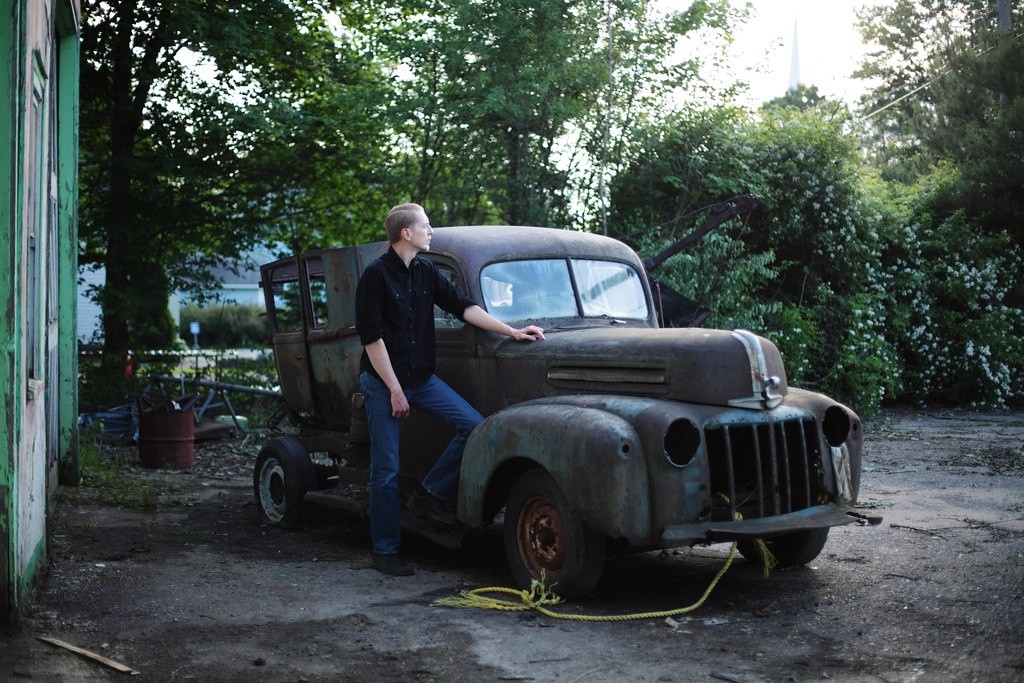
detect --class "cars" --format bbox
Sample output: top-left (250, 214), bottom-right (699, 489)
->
top-left (258, 221), bottom-right (863, 603)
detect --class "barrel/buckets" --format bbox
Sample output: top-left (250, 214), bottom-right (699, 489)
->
top-left (138, 407), bottom-right (194, 468)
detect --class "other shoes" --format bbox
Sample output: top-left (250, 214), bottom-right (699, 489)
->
top-left (372, 550), bottom-right (415, 577)
top-left (409, 490), bottom-right (458, 526)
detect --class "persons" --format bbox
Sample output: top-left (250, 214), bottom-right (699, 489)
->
top-left (354, 204), bottom-right (545, 577)
